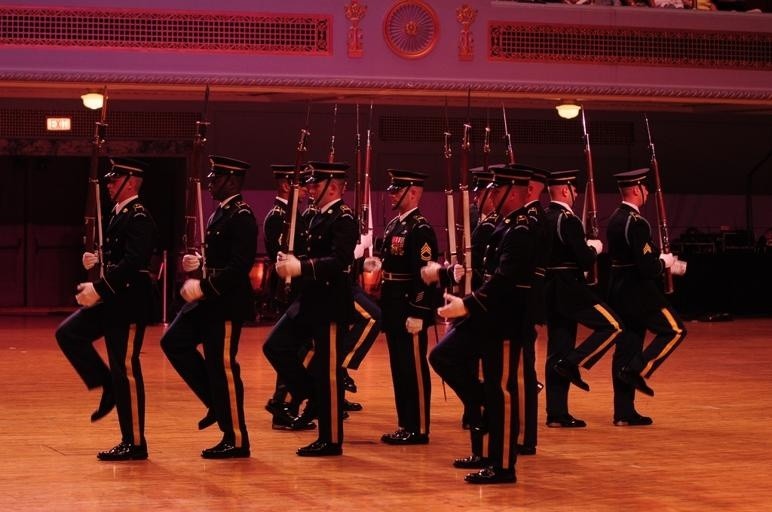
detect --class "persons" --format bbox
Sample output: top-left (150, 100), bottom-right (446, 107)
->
top-left (262, 160), bottom-right (381, 457)
top-left (54, 157), bottom-right (165, 461)
top-left (159, 154), bottom-right (259, 459)
top-left (400, 161), bottom-right (687, 483)
top-left (379, 167), bottom-right (438, 447)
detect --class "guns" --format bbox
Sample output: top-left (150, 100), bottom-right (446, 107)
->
top-left (443, 85), bottom-right (472, 323)
top-left (276, 104), bottom-right (311, 307)
top-left (183, 85), bottom-right (211, 300)
top-left (355, 102), bottom-right (374, 274)
top-left (327, 103), bottom-right (338, 164)
top-left (580, 103), bottom-right (600, 286)
top-left (643, 113), bottom-right (676, 295)
top-left (502, 99), bottom-right (516, 166)
top-left (482, 109), bottom-right (492, 171)
top-left (86, 84), bottom-right (108, 304)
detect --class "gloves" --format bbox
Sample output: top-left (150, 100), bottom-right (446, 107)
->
top-left (82, 251), bottom-right (100, 270)
top-left (354, 244), bottom-right (364, 259)
top-left (656, 252), bottom-right (688, 276)
top-left (453, 263), bottom-right (464, 283)
top-left (438, 293), bottom-right (466, 318)
top-left (77, 281), bottom-right (100, 307)
top-left (274, 251), bottom-right (303, 279)
top-left (181, 254), bottom-right (201, 272)
top-left (586, 239), bottom-right (603, 255)
top-left (181, 278), bottom-right (203, 302)
top-left (363, 256), bottom-right (383, 272)
top-left (420, 261), bottom-right (441, 285)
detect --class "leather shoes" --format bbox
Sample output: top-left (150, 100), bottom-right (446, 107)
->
top-left (613, 364), bottom-right (656, 427)
top-left (199, 441), bottom-right (251, 461)
top-left (89, 378), bottom-right (133, 423)
top-left (452, 382), bottom-right (543, 484)
top-left (97, 441), bottom-right (149, 463)
top-left (195, 399), bottom-right (232, 428)
top-left (379, 428), bottom-right (432, 446)
top-left (544, 351), bottom-right (589, 429)
top-left (265, 368), bottom-right (366, 458)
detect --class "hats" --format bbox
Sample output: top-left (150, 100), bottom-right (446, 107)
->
top-left (467, 163), bottom-right (583, 191)
top-left (270, 160), bottom-right (352, 185)
top-left (386, 166), bottom-right (428, 194)
top-left (102, 155), bottom-right (148, 181)
top-left (613, 167), bottom-right (651, 189)
top-left (204, 154), bottom-right (250, 179)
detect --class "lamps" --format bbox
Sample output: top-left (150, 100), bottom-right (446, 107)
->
top-left (554, 98), bottom-right (582, 122)
top-left (79, 90), bottom-right (103, 111)
